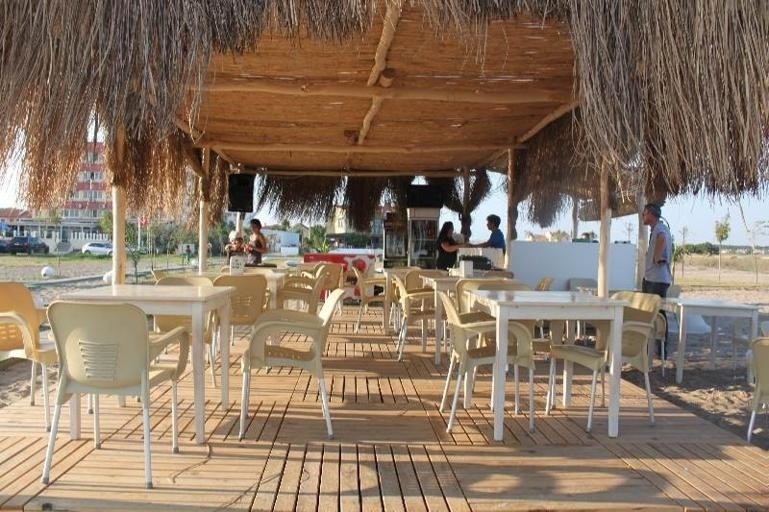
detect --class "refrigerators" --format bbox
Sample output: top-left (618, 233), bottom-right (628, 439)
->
top-left (383, 219), bottom-right (406, 268)
top-left (406, 206), bottom-right (439, 269)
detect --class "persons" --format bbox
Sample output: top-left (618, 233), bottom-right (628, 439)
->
top-left (185, 245), bottom-right (192, 263)
top-left (223, 229), bottom-right (248, 266)
top-left (466, 213), bottom-right (506, 254)
top-left (242, 218), bottom-right (268, 266)
top-left (434, 222), bottom-right (472, 270)
top-left (640, 203), bottom-right (673, 360)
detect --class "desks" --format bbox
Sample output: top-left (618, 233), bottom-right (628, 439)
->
top-left (660, 293), bottom-right (763, 386)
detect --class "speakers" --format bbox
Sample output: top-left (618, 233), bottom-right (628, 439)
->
top-left (227, 173), bottom-right (254, 212)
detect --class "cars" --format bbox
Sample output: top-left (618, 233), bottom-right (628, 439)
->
top-left (0, 240), bottom-right (11, 253)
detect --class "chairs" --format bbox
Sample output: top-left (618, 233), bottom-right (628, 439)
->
top-left (746, 336), bottom-right (768, 444)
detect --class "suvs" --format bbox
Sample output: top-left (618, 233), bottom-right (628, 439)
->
top-left (82, 241), bottom-right (113, 257)
top-left (10, 236), bottom-right (49, 256)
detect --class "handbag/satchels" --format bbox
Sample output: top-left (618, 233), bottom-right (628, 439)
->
top-left (459, 254), bottom-right (491, 270)
